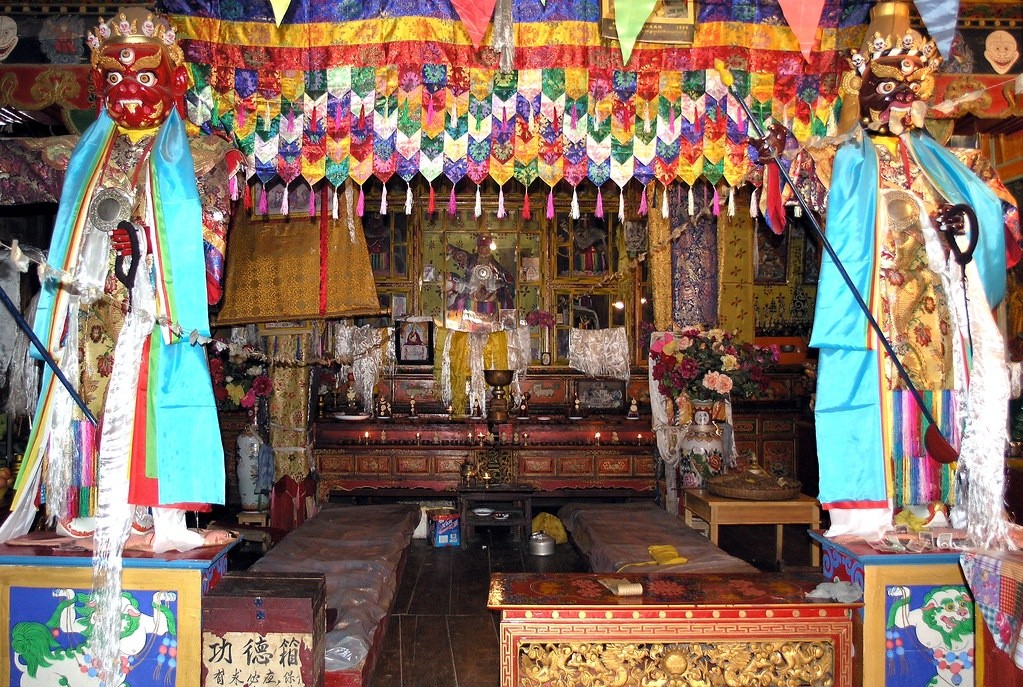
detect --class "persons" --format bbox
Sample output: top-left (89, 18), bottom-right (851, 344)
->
top-left (0, 13), bottom-right (243, 533)
top-left (440, 231), bottom-right (515, 315)
top-left (743, 30), bottom-right (1023, 531)
top-left (404, 328), bottom-right (423, 345)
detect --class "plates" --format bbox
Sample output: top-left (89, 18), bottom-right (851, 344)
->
top-left (494, 514), bottom-right (507, 519)
top-left (471, 507), bottom-right (495, 516)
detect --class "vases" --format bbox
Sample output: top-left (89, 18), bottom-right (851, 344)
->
top-left (234, 397), bottom-right (275, 512)
top-left (675, 401), bottom-right (725, 514)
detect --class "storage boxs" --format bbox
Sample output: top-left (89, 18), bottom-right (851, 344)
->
top-left (426, 509), bottom-right (460, 548)
top-left (202, 571), bottom-right (327, 687)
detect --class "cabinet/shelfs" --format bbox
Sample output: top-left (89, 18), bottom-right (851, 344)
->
top-left (316, 414), bottom-right (662, 507)
top-left (808, 527), bottom-right (1023, 687)
top-left (457, 483), bottom-right (534, 547)
top-left (0, 533), bottom-right (243, 687)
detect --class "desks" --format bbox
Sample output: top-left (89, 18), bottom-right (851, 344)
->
top-left (685, 488), bottom-right (820, 567)
top-left (485, 568), bottom-right (865, 687)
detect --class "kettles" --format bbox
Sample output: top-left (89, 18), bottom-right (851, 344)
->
top-left (529, 530), bottom-right (556, 555)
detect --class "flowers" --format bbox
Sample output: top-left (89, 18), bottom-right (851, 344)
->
top-left (523, 310), bottom-right (554, 328)
top-left (208, 337), bottom-right (272, 409)
top-left (650, 330), bottom-right (780, 408)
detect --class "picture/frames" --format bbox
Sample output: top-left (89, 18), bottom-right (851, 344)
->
top-left (750, 217), bottom-right (791, 286)
top-left (395, 318), bottom-right (436, 365)
top-left (257, 320), bottom-right (311, 336)
top-left (803, 225), bottom-right (821, 286)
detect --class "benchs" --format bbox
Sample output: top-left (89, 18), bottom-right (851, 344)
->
top-left (248, 501), bottom-right (421, 687)
top-left (558, 498), bottom-right (760, 573)
